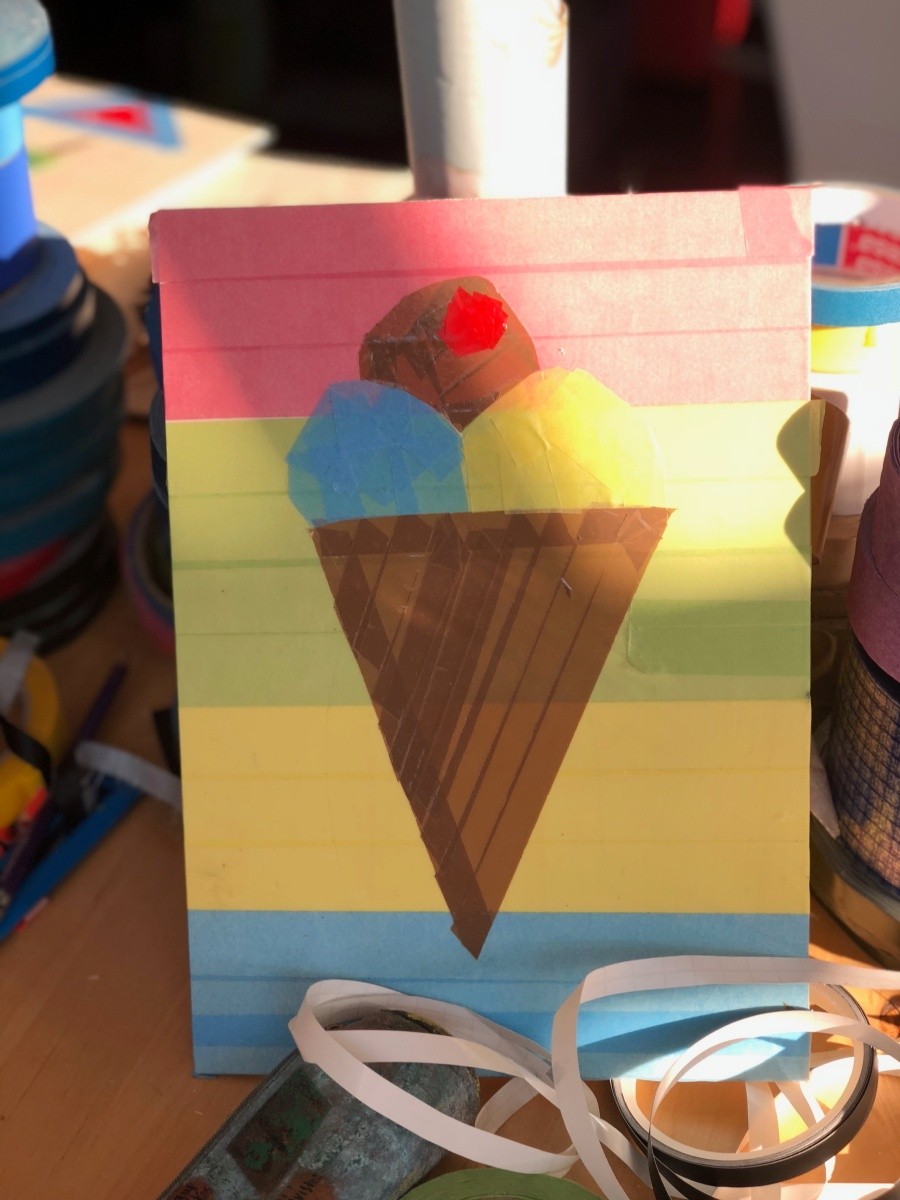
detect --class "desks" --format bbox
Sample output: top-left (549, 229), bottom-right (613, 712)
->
top-left (0, 106), bottom-right (410, 1200)
